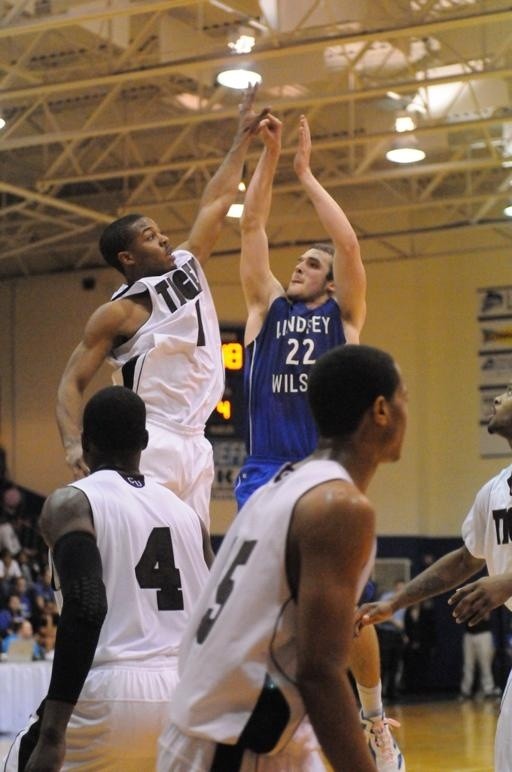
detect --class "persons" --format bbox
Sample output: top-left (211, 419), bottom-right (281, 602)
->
top-left (234, 112), bottom-right (406, 772)
top-left (0, 385), bottom-right (219, 772)
top-left (55, 80), bottom-right (270, 538)
top-left (352, 385), bottom-right (512, 772)
top-left (347, 549), bottom-right (512, 704)
top-left (0, 486), bottom-right (60, 661)
top-left (155, 345), bottom-right (409, 772)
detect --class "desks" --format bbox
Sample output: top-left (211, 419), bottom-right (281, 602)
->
top-left (1, 658), bottom-right (54, 735)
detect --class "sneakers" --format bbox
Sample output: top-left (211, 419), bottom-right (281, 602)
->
top-left (358, 707), bottom-right (407, 772)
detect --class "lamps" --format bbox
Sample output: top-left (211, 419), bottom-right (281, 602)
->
top-left (377, 94), bottom-right (428, 167)
top-left (225, 178), bottom-right (251, 219)
top-left (213, 23), bottom-right (264, 92)
